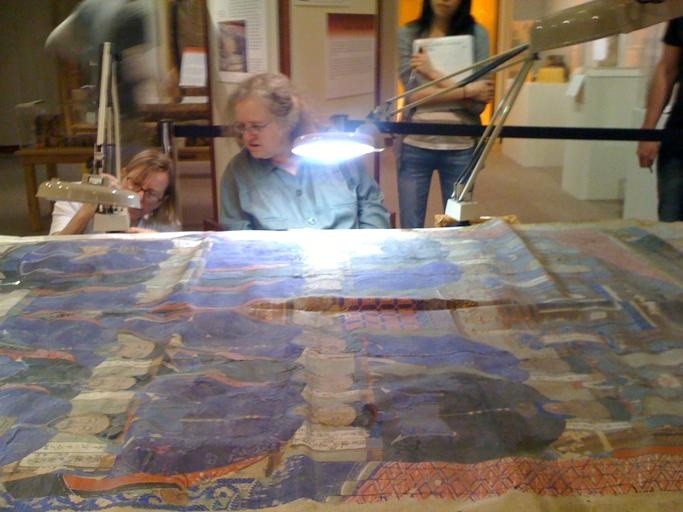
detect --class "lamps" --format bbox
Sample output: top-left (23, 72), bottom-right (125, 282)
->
top-left (523, 0), bottom-right (681, 54)
top-left (283, 41), bottom-right (550, 229)
top-left (29, 38), bottom-right (145, 238)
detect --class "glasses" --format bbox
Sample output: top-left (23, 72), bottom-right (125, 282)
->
top-left (125, 171), bottom-right (167, 205)
top-left (233, 114), bottom-right (278, 133)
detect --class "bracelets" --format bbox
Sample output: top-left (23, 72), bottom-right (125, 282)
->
top-left (464, 85), bottom-right (466, 100)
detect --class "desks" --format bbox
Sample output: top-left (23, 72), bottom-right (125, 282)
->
top-left (0, 220), bottom-right (682, 512)
top-left (11, 120), bottom-right (162, 241)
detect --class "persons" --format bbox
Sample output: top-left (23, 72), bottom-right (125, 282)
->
top-left (47, 148), bottom-right (183, 235)
top-left (394, 1), bottom-right (496, 228)
top-left (637, 18), bottom-right (683, 222)
top-left (217, 70), bottom-right (391, 229)
